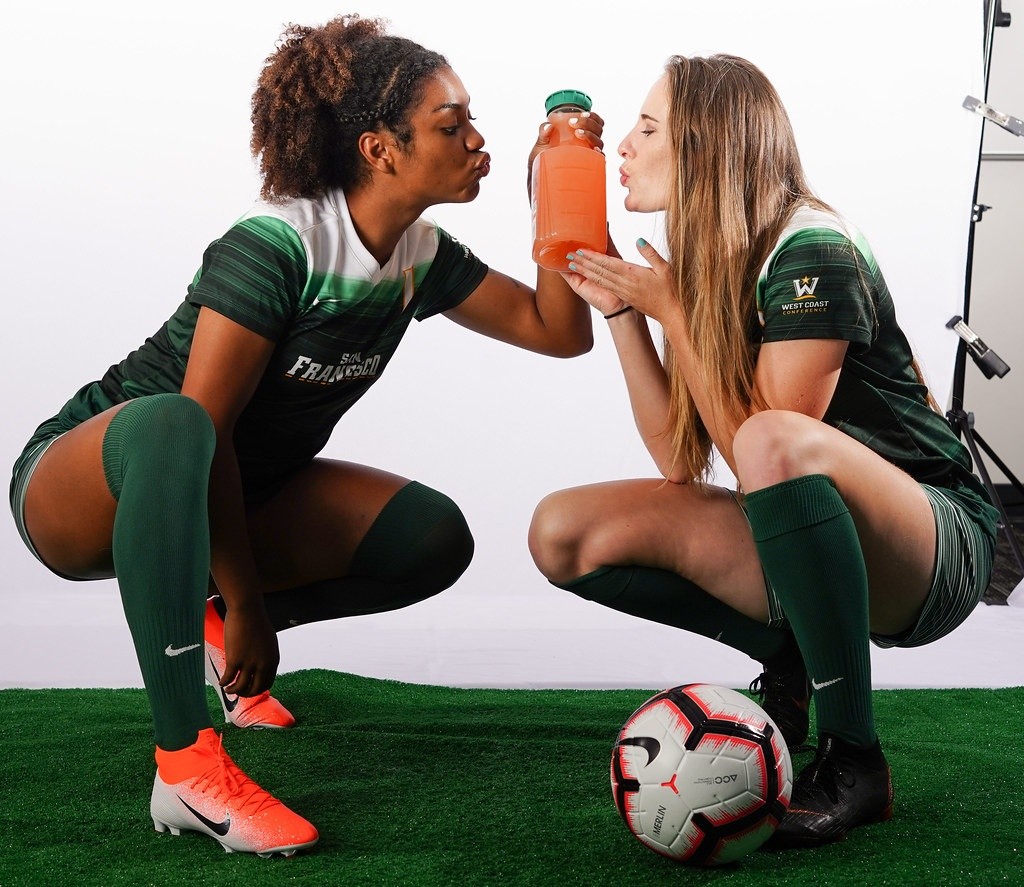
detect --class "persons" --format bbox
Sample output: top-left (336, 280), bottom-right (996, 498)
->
top-left (529, 55), bottom-right (997, 851)
top-left (9, 12), bottom-right (603, 858)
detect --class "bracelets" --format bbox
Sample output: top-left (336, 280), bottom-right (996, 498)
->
top-left (604, 305), bottom-right (633, 319)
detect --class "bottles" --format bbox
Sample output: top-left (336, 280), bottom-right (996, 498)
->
top-left (530, 89), bottom-right (609, 273)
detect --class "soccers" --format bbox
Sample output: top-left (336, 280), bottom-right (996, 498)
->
top-left (610, 684), bottom-right (793, 867)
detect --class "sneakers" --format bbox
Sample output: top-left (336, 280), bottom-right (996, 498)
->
top-left (148, 728), bottom-right (320, 861)
top-left (205, 594), bottom-right (297, 731)
top-left (765, 732), bottom-right (897, 843)
top-left (748, 660), bottom-right (812, 749)
